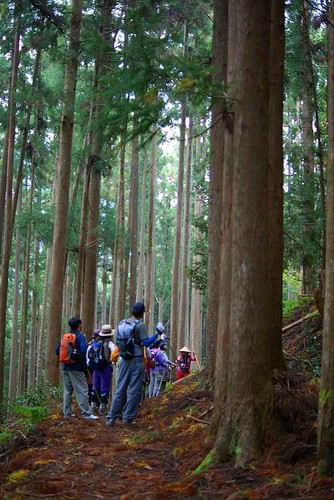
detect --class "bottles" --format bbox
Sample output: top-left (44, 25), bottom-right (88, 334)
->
top-left (72, 349), bottom-right (77, 354)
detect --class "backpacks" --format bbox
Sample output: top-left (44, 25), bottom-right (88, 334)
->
top-left (59, 332), bottom-right (81, 364)
top-left (88, 339), bottom-right (110, 370)
top-left (117, 319), bottom-right (139, 360)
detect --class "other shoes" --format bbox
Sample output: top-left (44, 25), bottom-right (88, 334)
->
top-left (122, 420), bottom-right (138, 426)
top-left (64, 414), bottom-right (75, 418)
top-left (84, 414), bottom-right (99, 420)
top-left (99, 409), bottom-right (107, 415)
top-left (90, 407), bottom-right (96, 413)
top-left (106, 419), bottom-right (114, 426)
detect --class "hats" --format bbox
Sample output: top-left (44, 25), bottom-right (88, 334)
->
top-left (98, 324), bottom-right (116, 337)
top-left (133, 302), bottom-right (146, 313)
top-left (91, 329), bottom-right (100, 338)
top-left (179, 346), bottom-right (191, 353)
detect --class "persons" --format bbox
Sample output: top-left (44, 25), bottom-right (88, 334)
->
top-left (56, 302), bottom-right (197, 426)
top-left (174, 346), bottom-right (196, 381)
top-left (56, 317), bottom-right (100, 419)
top-left (148, 341), bottom-right (160, 398)
top-left (104, 302), bottom-right (159, 426)
top-left (151, 343), bottom-right (176, 393)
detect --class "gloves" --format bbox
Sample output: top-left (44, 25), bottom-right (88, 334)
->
top-left (160, 334), bottom-right (167, 340)
top-left (156, 323), bottom-right (165, 334)
